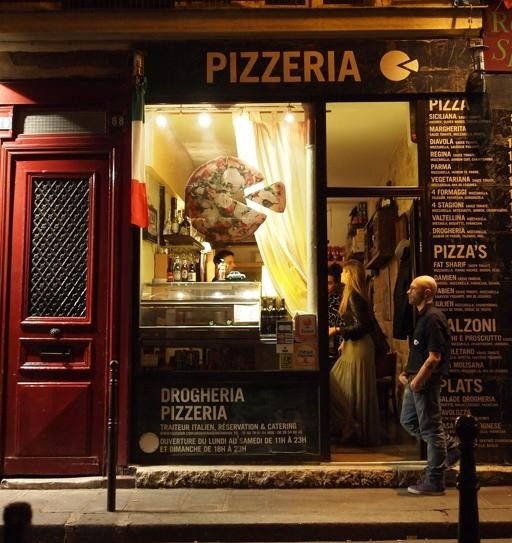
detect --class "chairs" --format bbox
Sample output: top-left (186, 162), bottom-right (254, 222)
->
top-left (377, 352), bottom-right (404, 445)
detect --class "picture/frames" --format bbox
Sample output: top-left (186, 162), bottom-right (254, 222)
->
top-left (143, 206), bottom-right (157, 244)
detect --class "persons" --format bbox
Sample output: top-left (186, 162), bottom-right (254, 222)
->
top-left (212, 250), bottom-right (237, 281)
top-left (328, 259), bottom-right (380, 442)
top-left (399, 275), bottom-right (459, 495)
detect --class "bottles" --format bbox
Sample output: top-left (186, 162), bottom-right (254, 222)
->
top-left (167, 253), bottom-right (200, 282)
top-left (166, 209), bottom-right (191, 235)
top-left (260, 297), bottom-right (289, 334)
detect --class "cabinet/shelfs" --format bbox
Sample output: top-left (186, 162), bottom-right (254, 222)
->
top-left (141, 281), bottom-right (261, 372)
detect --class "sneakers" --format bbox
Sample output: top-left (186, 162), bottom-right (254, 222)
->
top-left (408, 481), bottom-right (445, 496)
top-left (447, 446), bottom-right (461, 466)
